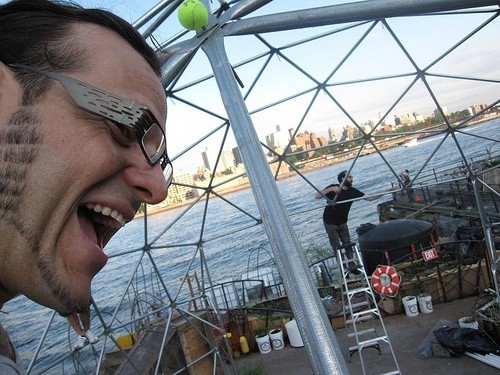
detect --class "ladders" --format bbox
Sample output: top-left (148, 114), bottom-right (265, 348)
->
top-left (335, 238), bottom-right (401, 375)
top-left (467, 160), bottom-right (500, 303)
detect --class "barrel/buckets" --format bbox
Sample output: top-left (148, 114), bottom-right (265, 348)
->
top-left (269, 328), bottom-right (285, 350)
top-left (417, 294), bottom-right (434, 314)
top-left (255, 333), bottom-right (272, 355)
top-left (402, 295), bottom-right (419, 317)
top-left (459, 316), bottom-right (479, 329)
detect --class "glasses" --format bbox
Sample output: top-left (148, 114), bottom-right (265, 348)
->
top-left (9, 63), bottom-right (174, 189)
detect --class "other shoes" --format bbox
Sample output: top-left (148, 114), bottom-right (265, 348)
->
top-left (74, 335), bottom-right (89, 349)
top-left (344, 272), bottom-right (350, 279)
top-left (85, 329), bottom-right (99, 344)
top-left (351, 269), bottom-right (361, 275)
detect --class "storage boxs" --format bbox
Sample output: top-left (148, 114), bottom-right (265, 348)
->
top-left (150, 310), bottom-right (224, 375)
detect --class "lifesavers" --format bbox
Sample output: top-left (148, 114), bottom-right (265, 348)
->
top-left (371, 266), bottom-right (400, 297)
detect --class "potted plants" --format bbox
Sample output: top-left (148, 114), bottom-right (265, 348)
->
top-left (402, 280), bottom-right (434, 316)
top-left (255, 326), bottom-right (285, 354)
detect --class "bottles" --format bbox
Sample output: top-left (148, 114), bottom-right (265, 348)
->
top-left (239, 336), bottom-right (249, 354)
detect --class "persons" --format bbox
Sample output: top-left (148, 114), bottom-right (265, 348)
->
top-left (57, 296), bottom-right (99, 353)
top-left (0, 0), bottom-right (174, 375)
top-left (315, 170), bottom-right (396, 279)
